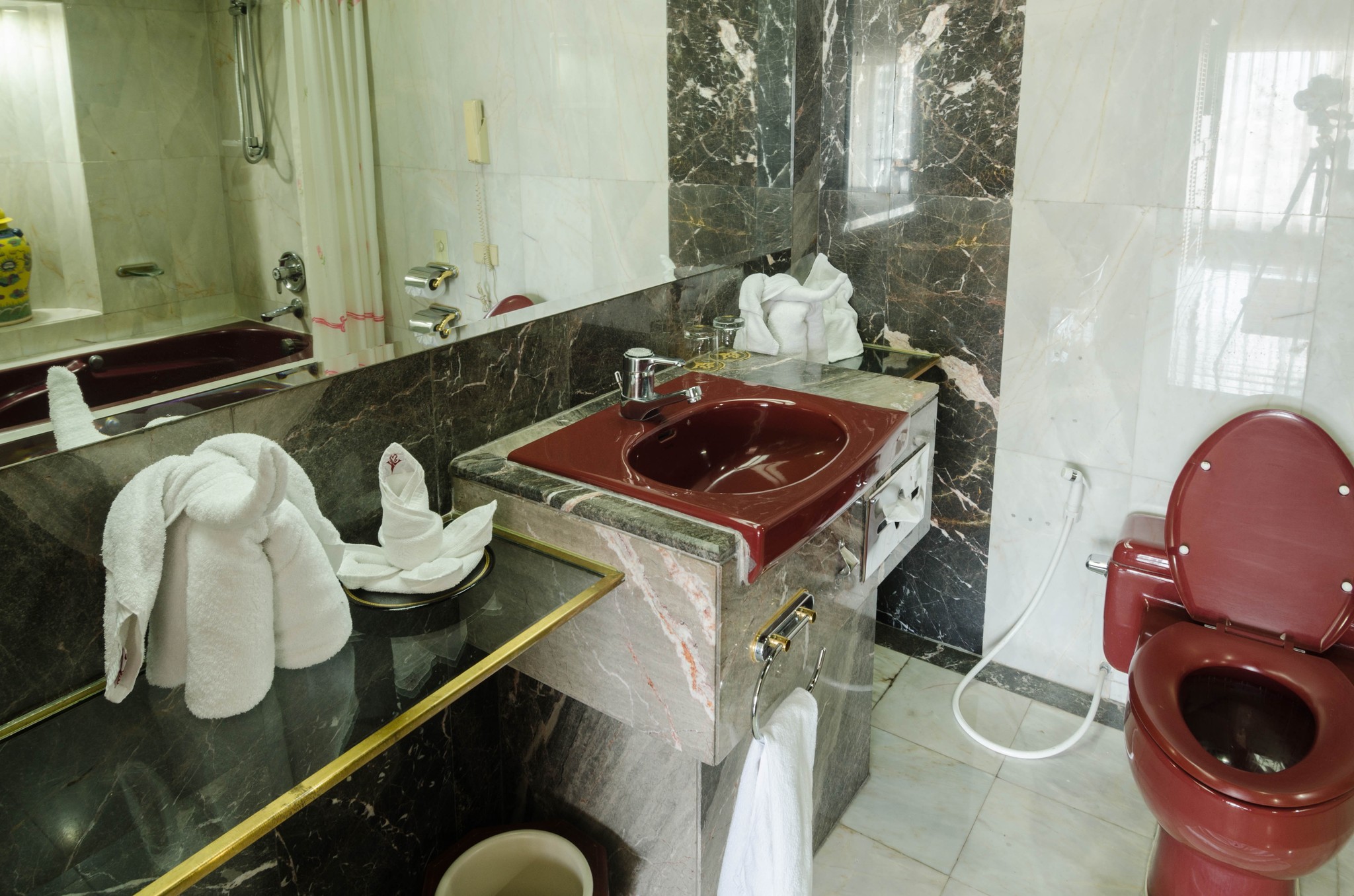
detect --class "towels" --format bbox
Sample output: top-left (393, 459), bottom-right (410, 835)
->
top-left (100, 431), bottom-right (357, 719)
top-left (731, 251), bottom-right (865, 363)
top-left (338, 442), bottom-right (498, 595)
top-left (41, 364), bottom-right (108, 453)
top-left (711, 687), bottom-right (819, 896)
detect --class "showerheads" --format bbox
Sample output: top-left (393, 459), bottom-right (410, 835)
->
top-left (1061, 467), bottom-right (1089, 520)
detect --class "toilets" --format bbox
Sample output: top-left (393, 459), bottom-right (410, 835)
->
top-left (1081, 407), bottom-right (1354, 896)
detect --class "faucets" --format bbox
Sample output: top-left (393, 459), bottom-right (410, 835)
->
top-left (614, 347), bottom-right (703, 422)
top-left (257, 299), bottom-right (304, 325)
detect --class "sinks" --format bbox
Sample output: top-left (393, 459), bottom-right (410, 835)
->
top-left (505, 370), bottom-right (913, 580)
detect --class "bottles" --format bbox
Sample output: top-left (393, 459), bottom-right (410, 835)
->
top-left (0, 207), bottom-right (33, 328)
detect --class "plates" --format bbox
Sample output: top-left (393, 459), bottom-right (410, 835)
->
top-left (340, 544), bottom-right (496, 610)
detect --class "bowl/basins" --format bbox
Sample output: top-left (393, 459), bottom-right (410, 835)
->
top-left (434, 828), bottom-right (594, 896)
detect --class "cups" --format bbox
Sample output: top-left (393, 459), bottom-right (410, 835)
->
top-left (683, 315), bottom-right (746, 371)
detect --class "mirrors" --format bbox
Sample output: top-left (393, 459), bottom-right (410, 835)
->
top-left (0, 0), bottom-right (796, 468)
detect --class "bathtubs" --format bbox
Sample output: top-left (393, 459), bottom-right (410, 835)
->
top-left (0, 314), bottom-right (320, 444)
